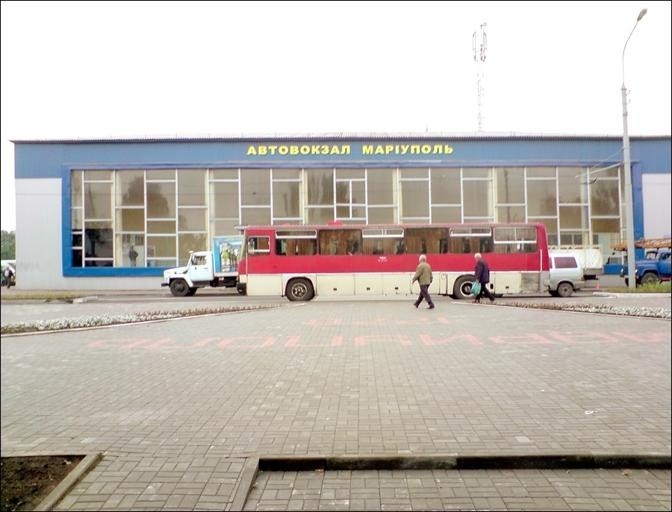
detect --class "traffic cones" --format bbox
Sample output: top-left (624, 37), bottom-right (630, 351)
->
top-left (595, 282), bottom-right (602, 290)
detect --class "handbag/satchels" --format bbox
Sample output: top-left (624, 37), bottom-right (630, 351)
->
top-left (471, 281), bottom-right (481, 294)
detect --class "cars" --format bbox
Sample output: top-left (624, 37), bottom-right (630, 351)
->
top-left (1, 260), bottom-right (16, 286)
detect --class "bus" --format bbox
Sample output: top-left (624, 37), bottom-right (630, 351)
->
top-left (236, 221), bottom-right (552, 304)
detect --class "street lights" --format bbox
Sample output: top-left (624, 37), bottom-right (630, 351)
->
top-left (620, 7), bottom-right (649, 290)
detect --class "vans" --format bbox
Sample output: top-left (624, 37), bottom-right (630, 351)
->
top-left (548, 255), bottom-right (584, 297)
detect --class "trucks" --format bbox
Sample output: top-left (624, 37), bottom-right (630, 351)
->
top-left (546, 244), bottom-right (602, 280)
top-left (162, 235), bottom-right (244, 297)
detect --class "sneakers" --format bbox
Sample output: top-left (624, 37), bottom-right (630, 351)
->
top-left (428, 305), bottom-right (434, 309)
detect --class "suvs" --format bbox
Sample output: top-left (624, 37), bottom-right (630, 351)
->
top-left (621, 251), bottom-right (671, 288)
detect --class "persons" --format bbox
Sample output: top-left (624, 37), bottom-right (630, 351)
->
top-left (129, 244), bottom-right (138, 266)
top-left (471, 251), bottom-right (497, 305)
top-left (409, 254), bottom-right (436, 309)
top-left (2, 266), bottom-right (15, 288)
